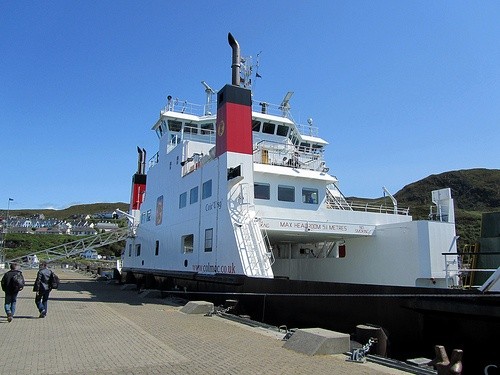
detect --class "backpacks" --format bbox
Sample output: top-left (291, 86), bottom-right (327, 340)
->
top-left (12, 271), bottom-right (24, 291)
top-left (48, 270), bottom-right (58, 289)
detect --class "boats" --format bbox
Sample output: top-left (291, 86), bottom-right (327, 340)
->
top-left (113, 31), bottom-right (500, 375)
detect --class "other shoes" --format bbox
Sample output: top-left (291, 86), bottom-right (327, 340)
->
top-left (43, 315), bottom-right (45, 318)
top-left (7, 313), bottom-right (12, 322)
top-left (39, 309), bottom-right (45, 317)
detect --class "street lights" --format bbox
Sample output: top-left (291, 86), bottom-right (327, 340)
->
top-left (5, 197), bottom-right (14, 232)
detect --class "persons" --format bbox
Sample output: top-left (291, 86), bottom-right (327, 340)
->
top-left (1, 263), bottom-right (24, 322)
top-left (33, 261), bottom-right (52, 317)
